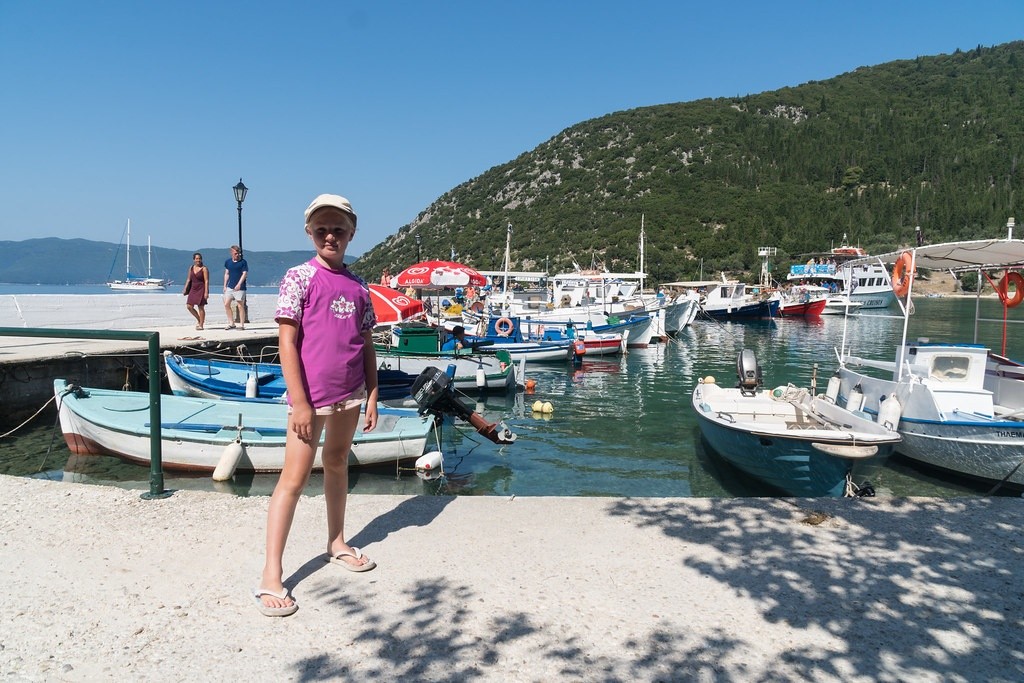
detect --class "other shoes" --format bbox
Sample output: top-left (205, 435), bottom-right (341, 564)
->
top-left (196, 327), bottom-right (204, 330)
top-left (196, 324), bottom-right (200, 327)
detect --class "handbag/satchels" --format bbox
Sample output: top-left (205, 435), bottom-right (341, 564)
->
top-left (186, 280), bottom-right (191, 294)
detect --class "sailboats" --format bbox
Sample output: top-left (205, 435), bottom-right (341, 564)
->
top-left (105, 218), bottom-right (175, 291)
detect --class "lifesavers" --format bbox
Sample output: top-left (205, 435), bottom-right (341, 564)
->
top-left (889, 251), bottom-right (916, 299)
top-left (495, 317), bottom-right (514, 337)
top-left (996, 272), bottom-right (1024, 308)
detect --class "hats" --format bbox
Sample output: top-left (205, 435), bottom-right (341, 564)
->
top-left (305, 194), bottom-right (357, 226)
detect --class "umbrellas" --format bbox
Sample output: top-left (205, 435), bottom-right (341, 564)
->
top-left (365, 282), bottom-right (428, 329)
top-left (390, 258), bottom-right (486, 352)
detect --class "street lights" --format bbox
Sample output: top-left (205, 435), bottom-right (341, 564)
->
top-left (414, 233), bottom-right (421, 264)
top-left (231, 177), bottom-right (250, 323)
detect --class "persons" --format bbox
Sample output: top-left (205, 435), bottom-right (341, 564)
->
top-left (441, 326), bottom-right (469, 352)
top-left (254, 194), bottom-right (377, 616)
top-left (381, 268), bottom-right (395, 288)
top-left (455, 285), bottom-right (487, 309)
top-left (223, 245), bottom-right (248, 330)
top-left (183, 253), bottom-right (209, 330)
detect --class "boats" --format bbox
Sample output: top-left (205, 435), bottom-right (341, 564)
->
top-left (163, 349), bottom-right (457, 416)
top-left (360, 210), bottom-right (897, 395)
top-left (689, 348), bottom-right (901, 499)
top-left (51, 366), bottom-right (520, 480)
top-left (821, 238), bottom-right (1024, 493)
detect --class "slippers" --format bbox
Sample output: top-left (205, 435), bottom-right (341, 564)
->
top-left (323, 547), bottom-right (376, 571)
top-left (249, 588), bottom-right (298, 616)
top-left (225, 325), bottom-right (236, 330)
